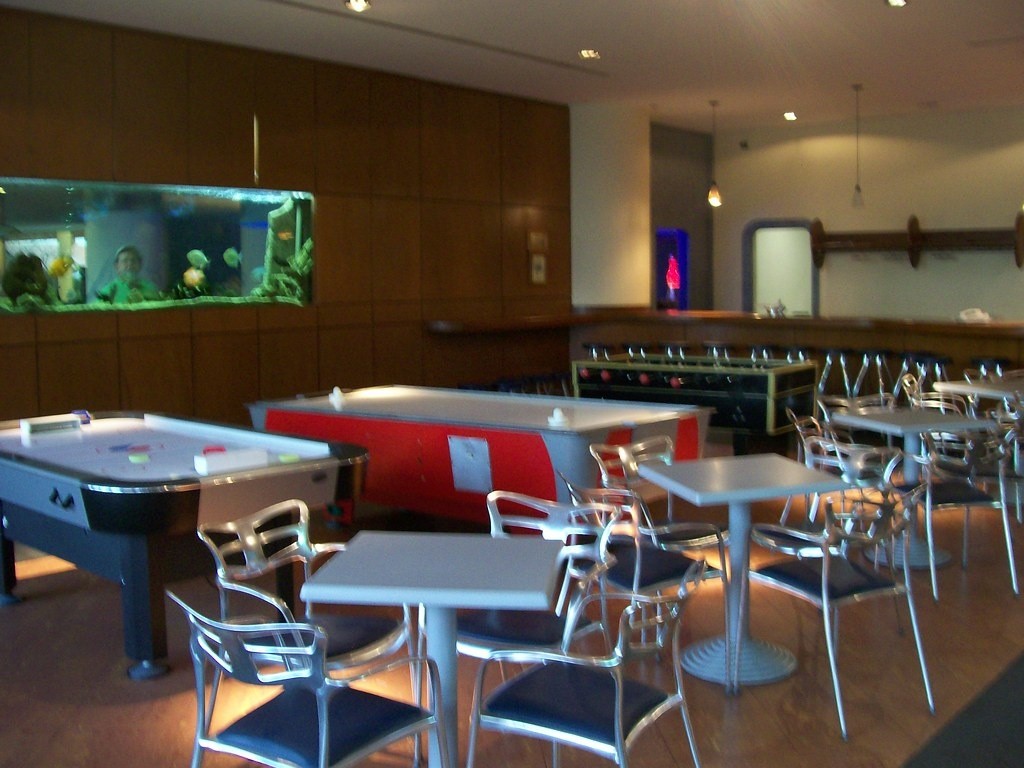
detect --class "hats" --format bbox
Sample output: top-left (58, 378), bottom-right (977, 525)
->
top-left (114, 244), bottom-right (141, 262)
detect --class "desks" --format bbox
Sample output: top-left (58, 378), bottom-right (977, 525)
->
top-left (637, 453), bottom-right (850, 687)
top-left (1, 410), bottom-right (368, 678)
top-left (832, 409), bottom-right (997, 570)
top-left (248, 383), bottom-right (716, 546)
top-left (570, 355), bottom-right (820, 459)
top-left (933, 379), bottom-right (1024, 504)
top-left (298, 530), bottom-right (564, 768)
top-left (421, 307), bottom-right (1023, 340)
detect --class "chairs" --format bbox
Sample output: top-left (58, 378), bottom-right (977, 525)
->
top-left (164, 369), bottom-right (1024, 768)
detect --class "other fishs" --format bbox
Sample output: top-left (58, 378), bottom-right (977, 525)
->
top-left (222, 247), bottom-right (243, 269)
top-left (48, 255), bottom-right (74, 279)
top-left (183, 249), bottom-right (212, 290)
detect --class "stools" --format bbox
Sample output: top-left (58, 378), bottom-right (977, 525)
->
top-left (498, 340), bottom-right (1012, 414)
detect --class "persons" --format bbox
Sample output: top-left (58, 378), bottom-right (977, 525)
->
top-left (0, 253), bottom-right (74, 306)
top-left (94, 245), bottom-right (163, 305)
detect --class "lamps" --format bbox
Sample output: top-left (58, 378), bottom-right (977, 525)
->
top-left (851, 83), bottom-right (864, 208)
top-left (708, 100), bottom-right (722, 207)
